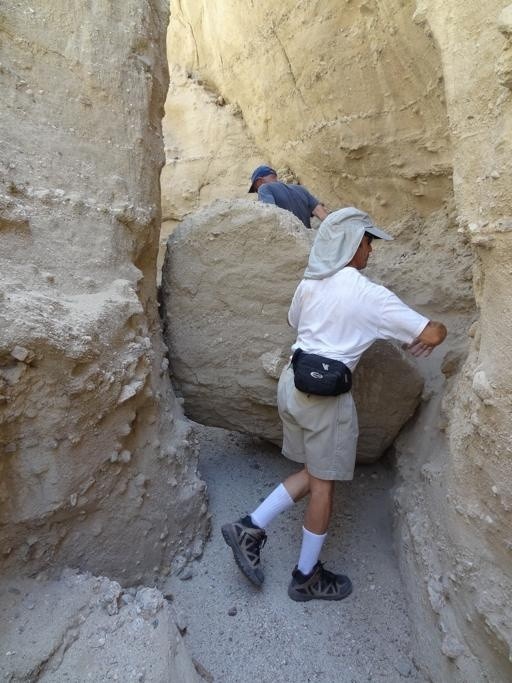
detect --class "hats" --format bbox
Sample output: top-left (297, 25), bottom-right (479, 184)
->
top-left (363, 207), bottom-right (396, 241)
top-left (247, 165), bottom-right (277, 193)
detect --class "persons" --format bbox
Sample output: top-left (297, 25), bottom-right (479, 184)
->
top-left (248, 165), bottom-right (328, 229)
top-left (222, 207), bottom-right (447, 602)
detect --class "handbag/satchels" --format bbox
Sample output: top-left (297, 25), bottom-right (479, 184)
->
top-left (293, 352), bottom-right (349, 396)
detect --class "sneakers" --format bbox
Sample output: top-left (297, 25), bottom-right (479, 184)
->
top-left (288, 561), bottom-right (352, 601)
top-left (221, 515), bottom-right (267, 587)
